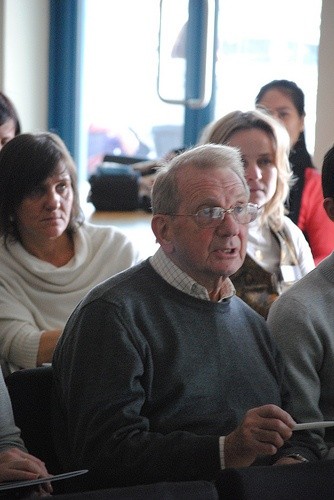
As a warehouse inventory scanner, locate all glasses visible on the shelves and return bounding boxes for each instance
[169,203,258,228]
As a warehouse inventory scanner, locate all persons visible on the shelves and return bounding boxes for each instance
[255,80,334,268]
[266,145,334,462]
[0,147,143,373]
[0,92,20,152]
[49,142,324,488]
[0,366,54,500]
[195,110,316,321]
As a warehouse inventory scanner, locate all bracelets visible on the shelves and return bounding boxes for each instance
[286,454,310,463]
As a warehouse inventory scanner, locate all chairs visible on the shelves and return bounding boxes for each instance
[5,369,334,500]
